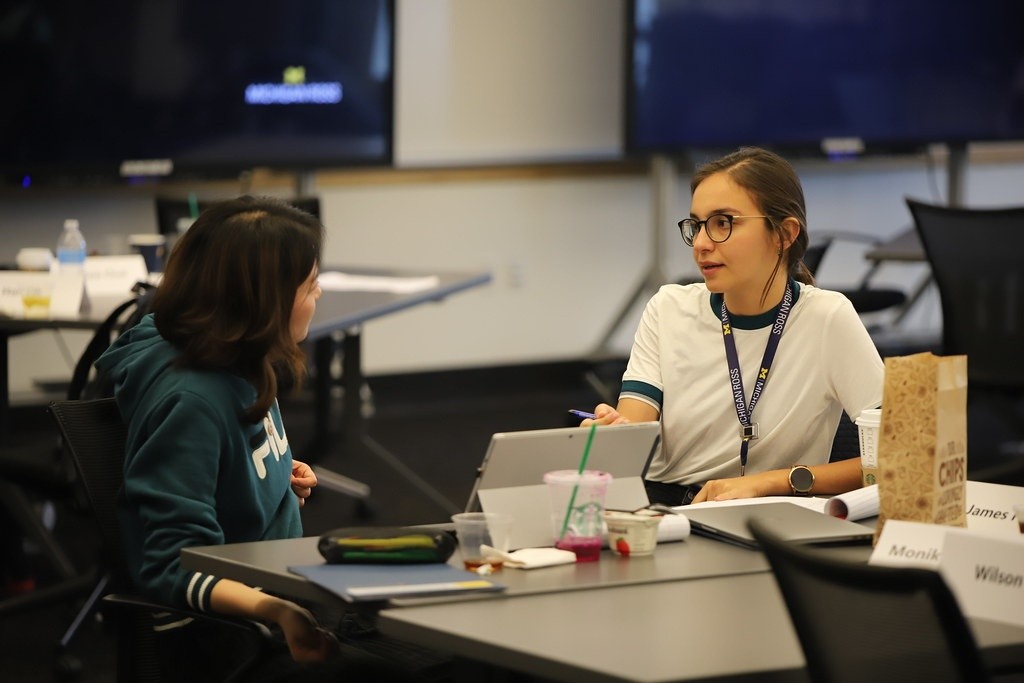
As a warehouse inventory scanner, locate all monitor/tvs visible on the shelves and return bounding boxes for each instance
[0,0,396,191]
[623,0,1024,171]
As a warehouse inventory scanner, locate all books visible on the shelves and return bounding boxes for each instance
[674,483,881,521]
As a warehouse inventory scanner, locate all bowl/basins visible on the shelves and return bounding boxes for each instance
[605,515,661,557]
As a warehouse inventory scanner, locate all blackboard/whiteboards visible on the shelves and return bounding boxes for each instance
[0,0,650,200]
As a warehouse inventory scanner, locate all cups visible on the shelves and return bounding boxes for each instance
[451,512,516,571]
[543,469,612,562]
[855,409,882,487]
[16,247,52,273]
[128,233,167,273]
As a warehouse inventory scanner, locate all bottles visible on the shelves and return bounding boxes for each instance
[57,218,85,274]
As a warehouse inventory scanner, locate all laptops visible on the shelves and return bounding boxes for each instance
[675,502,875,550]
[406,422,662,532]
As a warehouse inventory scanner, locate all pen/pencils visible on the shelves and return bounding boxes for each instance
[569,408,596,419]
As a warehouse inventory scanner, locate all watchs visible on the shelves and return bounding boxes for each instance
[789,464,815,497]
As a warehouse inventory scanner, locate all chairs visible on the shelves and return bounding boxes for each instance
[0,295,289,683]
[745,518,1024,683]
[575,230,910,405]
[902,194,1024,480]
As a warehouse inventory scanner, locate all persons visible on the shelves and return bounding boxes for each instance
[580,148,885,505]
[60,196,456,683]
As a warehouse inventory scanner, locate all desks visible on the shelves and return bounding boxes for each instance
[185,523,1023,683]
[862,232,941,334]
[1,249,491,472]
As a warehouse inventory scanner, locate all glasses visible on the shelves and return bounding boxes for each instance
[678,212,772,246]
